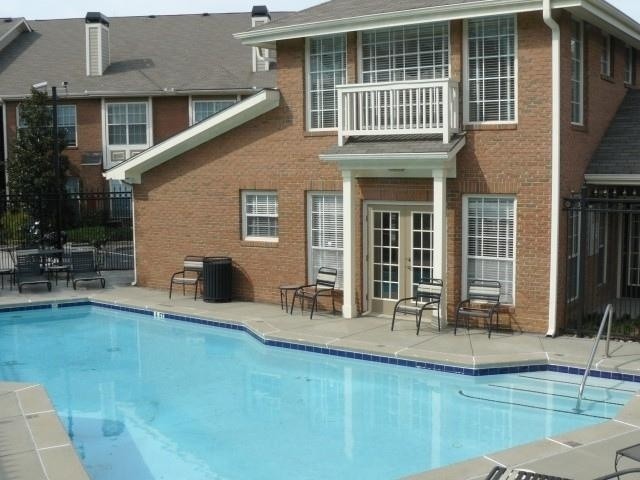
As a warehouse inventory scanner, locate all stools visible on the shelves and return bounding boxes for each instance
[278,284,307,312]
[610,441,640,480]
[483,463,570,479]
[47,263,71,289]
[0,268,14,291]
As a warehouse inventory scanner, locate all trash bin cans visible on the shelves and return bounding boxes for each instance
[203,257,232,303]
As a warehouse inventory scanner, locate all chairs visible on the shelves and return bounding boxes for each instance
[69,251,107,290]
[11,254,53,293]
[453,279,503,339]
[390,274,443,337]
[290,265,340,318]
[168,255,204,301]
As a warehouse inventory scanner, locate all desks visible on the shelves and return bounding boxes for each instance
[18,248,65,276]
